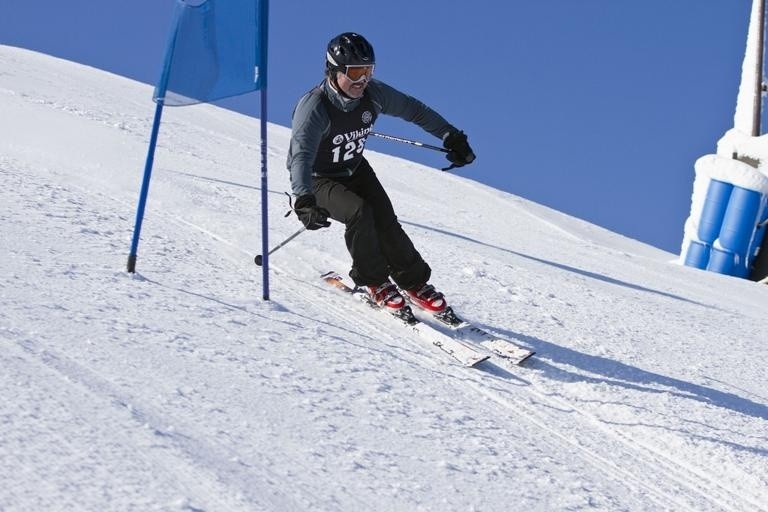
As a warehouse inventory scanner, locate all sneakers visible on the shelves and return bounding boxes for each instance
[367,282,406,310]
[402,282,447,311]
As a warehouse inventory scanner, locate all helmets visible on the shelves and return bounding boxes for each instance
[327,32,376,65]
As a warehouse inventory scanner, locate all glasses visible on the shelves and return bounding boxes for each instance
[345,65,374,83]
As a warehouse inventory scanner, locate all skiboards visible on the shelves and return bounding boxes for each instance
[314,258,536,368]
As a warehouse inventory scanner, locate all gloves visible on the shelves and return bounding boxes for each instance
[293,193,332,231]
[443,129,476,170]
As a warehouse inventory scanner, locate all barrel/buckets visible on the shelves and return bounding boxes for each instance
[706,245,754,278]
[683,239,712,269]
[698,176,735,241]
[718,185,768,257]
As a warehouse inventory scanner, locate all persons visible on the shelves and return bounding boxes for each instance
[286,32,475,314]
